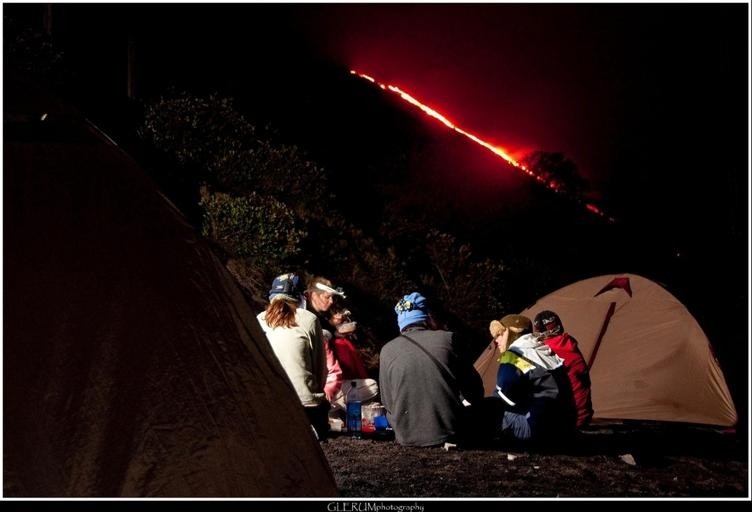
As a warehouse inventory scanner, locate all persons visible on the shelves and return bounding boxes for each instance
[378,291,485,450]
[465,316,575,454]
[256,274,332,440]
[531,311,595,429]
[301,275,355,332]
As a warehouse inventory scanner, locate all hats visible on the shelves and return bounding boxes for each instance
[534,309,564,336]
[393,290,432,330]
[488,314,533,350]
[266,271,304,306]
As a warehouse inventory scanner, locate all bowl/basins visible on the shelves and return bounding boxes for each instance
[337,322,357,333]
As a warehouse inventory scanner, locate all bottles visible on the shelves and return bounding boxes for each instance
[346,381,361,438]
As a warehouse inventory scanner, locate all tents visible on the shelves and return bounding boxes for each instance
[460,273,738,427]
